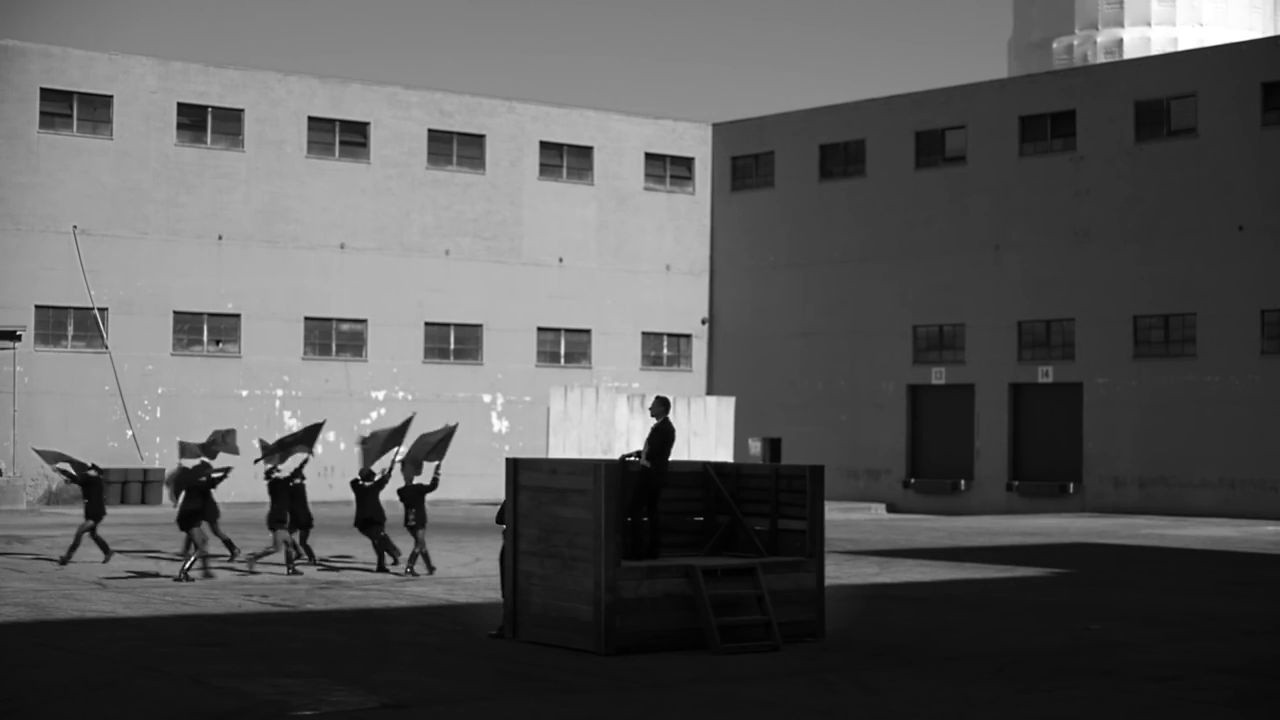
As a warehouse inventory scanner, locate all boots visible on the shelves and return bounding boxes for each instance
[178,555,197,582]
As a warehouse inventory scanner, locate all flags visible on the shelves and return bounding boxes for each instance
[165,466,229,504]
[253,422,323,464]
[180,428,240,459]
[359,416,414,468]
[398,425,457,476]
[35,449,88,474]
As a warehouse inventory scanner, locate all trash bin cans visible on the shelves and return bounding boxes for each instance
[101,468,126,505]
[143,468,165,505]
[123,468,144,505]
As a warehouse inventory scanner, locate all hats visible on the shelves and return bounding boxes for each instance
[358,467,375,482]
[264,466,279,475]
[88,462,104,474]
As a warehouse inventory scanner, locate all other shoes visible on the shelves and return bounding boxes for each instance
[101,550,114,563]
[376,567,390,573]
[287,567,304,576]
[428,566,436,576]
[228,548,240,561]
[392,561,399,566]
[405,567,419,577]
[202,570,213,578]
[246,554,255,571]
[60,555,67,564]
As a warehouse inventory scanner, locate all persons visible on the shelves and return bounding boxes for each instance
[350,468,401,572]
[488,500,506,638]
[620,396,674,560]
[245,457,316,575]
[59,463,113,565]
[176,460,241,582]
[396,462,440,576]
